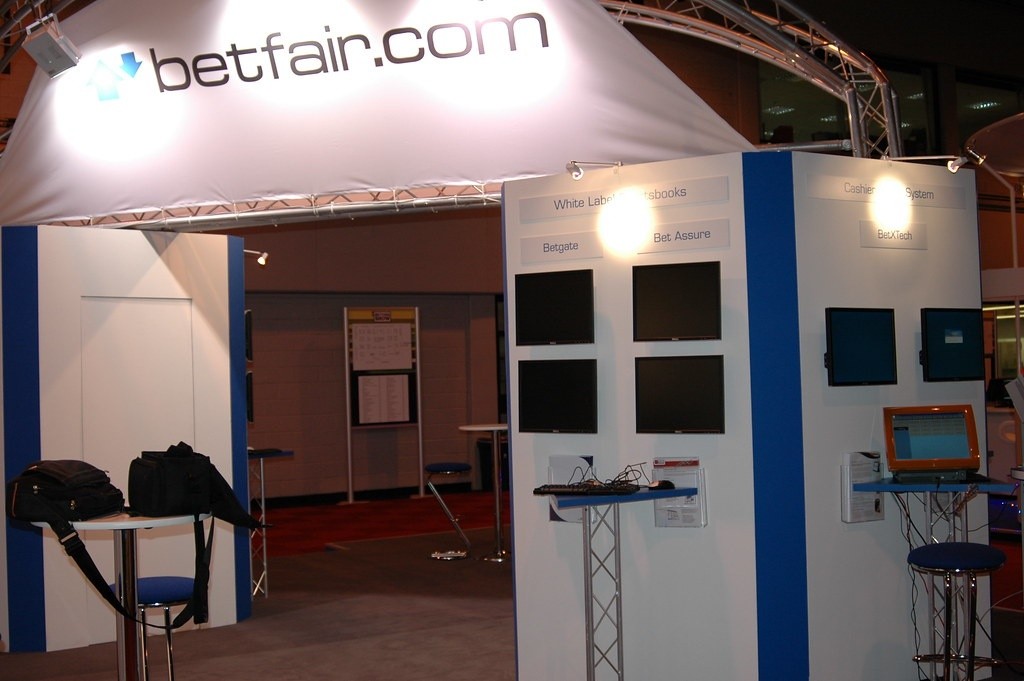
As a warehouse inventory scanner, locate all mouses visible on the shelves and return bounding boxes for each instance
[647,480,675,490]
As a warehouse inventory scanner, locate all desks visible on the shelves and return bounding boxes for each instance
[249,448,295,600]
[458,423,509,562]
[32,513,214,681]
[553,485,698,681]
[855,475,1016,681]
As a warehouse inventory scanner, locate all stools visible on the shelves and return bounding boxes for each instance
[422,462,472,559]
[907,541,1007,681]
[108,575,195,681]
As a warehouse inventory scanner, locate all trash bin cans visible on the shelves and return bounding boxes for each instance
[478,437,510,492]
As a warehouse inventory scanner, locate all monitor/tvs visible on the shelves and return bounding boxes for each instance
[635,355,725,435]
[632,261,722,341]
[515,269,595,346]
[986,378,1017,408]
[518,359,598,434]
[883,404,980,485]
[920,308,985,382]
[825,306,898,386]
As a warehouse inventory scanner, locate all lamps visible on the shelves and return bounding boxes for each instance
[565,161,623,180]
[23,13,83,79]
[884,148,968,173]
[243,250,269,266]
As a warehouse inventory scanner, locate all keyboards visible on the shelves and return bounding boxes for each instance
[533,484,641,495]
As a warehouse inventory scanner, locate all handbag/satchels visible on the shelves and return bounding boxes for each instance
[129,441,272,531]
[9,458,124,525]
